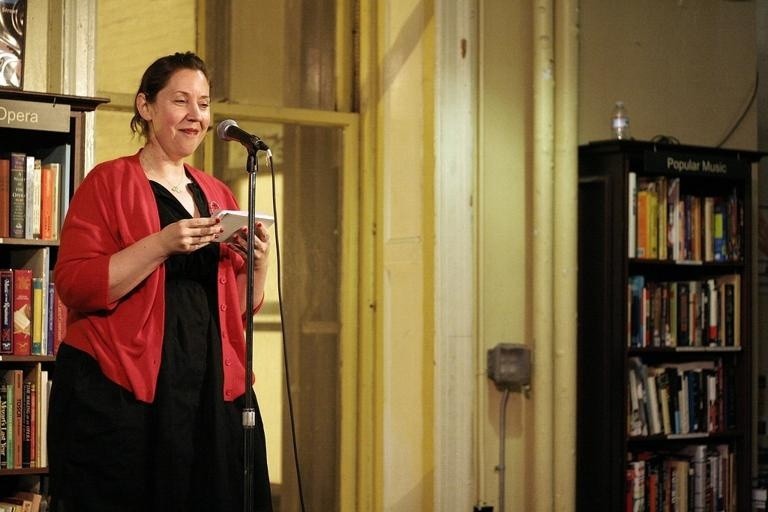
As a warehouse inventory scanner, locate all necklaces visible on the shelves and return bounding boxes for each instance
[141,150,185,193]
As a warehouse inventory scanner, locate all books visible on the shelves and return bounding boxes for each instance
[627,356,739,437]
[627,274,741,349]
[0,144,72,512]
[626,444,738,511]
[628,172,745,263]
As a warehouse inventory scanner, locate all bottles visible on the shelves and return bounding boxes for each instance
[610,101,630,139]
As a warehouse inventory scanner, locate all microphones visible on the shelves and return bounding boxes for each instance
[213,118,270,152]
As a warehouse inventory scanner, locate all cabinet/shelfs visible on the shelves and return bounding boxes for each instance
[578,139,764,512]
[0,88,109,511]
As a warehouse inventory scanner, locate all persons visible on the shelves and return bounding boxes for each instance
[46,51,271,512]
